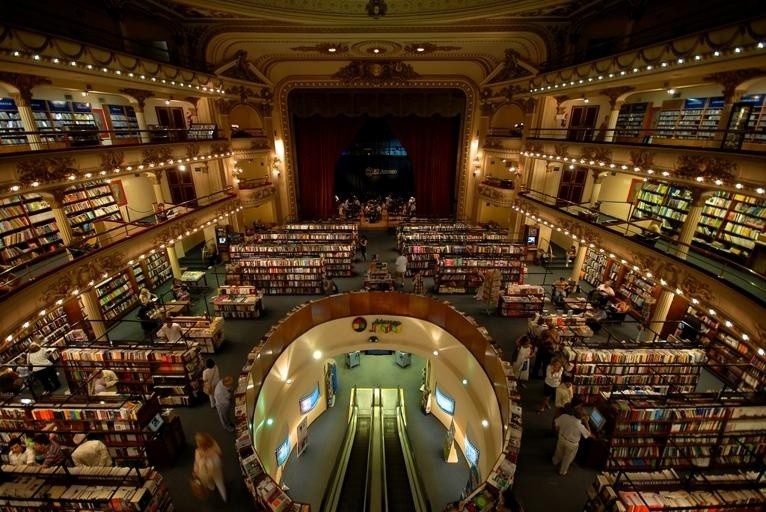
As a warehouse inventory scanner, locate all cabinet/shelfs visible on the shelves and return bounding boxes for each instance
[0,95,141,154]
[0,182,128,277]
[635,181,765,272]
[612,95,766,153]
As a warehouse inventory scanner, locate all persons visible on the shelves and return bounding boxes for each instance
[354,194,400,226]
[358,234,369,264]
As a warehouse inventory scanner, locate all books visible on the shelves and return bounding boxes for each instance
[1,102,357,511]
[396,93,765,512]
[361,252,394,293]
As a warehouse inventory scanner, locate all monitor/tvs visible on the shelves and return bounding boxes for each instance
[589,406,607,433]
